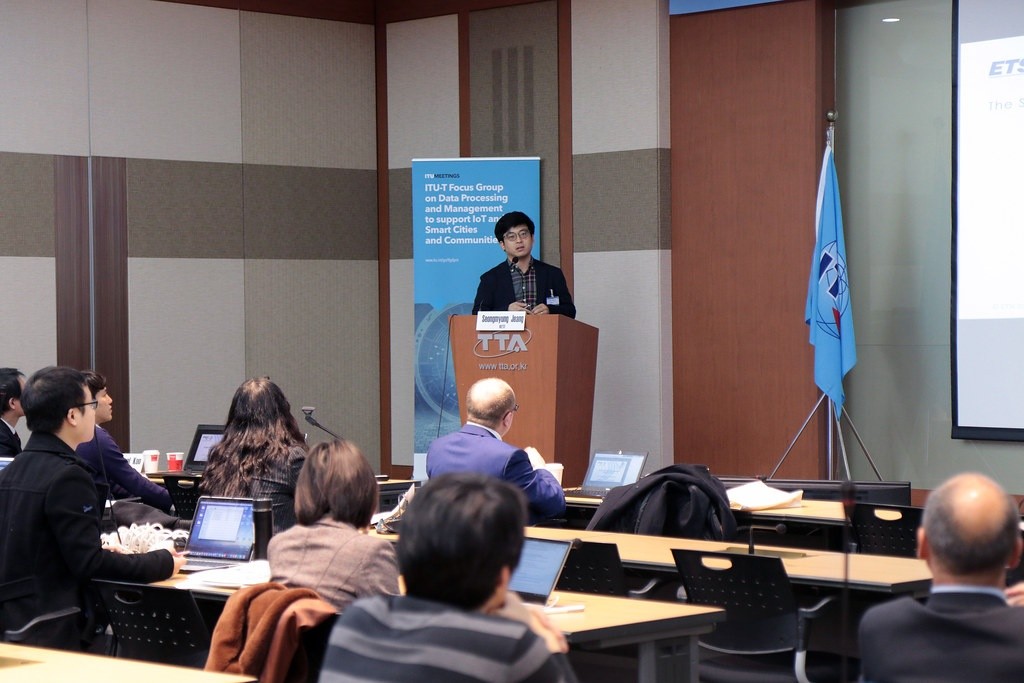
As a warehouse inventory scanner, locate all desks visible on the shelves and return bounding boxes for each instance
[105,538,724,683]
[378,525,933,683]
[0,642,259,683]
[147,471,421,510]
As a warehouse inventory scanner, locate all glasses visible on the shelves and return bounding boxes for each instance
[502,229,531,241]
[502,404,519,421]
[65,398,98,418]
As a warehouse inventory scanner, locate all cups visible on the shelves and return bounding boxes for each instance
[166,452,184,471]
[142,449,160,473]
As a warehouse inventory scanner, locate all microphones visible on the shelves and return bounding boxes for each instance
[479,257,519,311]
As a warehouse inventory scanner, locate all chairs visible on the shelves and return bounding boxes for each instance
[91,579,211,671]
[843,501,925,559]
[555,541,658,683]
[161,476,202,520]
[670,548,834,683]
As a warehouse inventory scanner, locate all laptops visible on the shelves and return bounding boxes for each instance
[174,496,257,572]
[563,451,650,499]
[506,537,573,606]
[183,424,229,477]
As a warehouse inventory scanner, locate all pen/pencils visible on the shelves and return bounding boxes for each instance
[177,551,190,556]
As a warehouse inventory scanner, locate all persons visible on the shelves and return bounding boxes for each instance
[472,211,577,319]
[317,473,577,683]
[0,366,187,651]
[199,377,309,538]
[857,472,1024,683]
[267,440,402,613]
[426,378,566,526]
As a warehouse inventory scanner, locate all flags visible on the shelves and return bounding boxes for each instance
[804,145,857,422]
